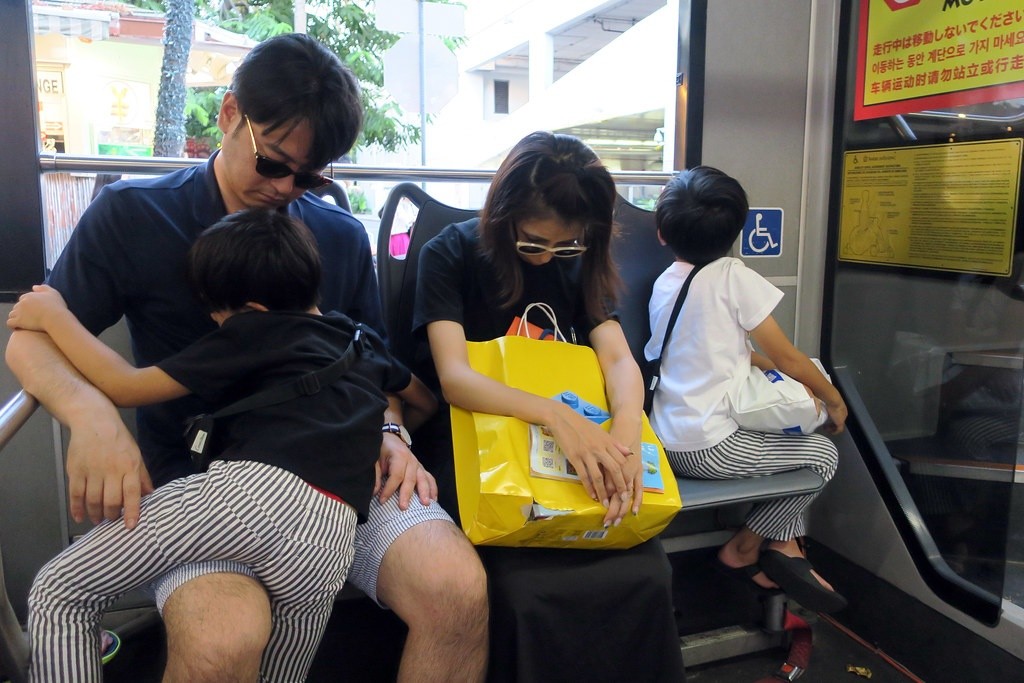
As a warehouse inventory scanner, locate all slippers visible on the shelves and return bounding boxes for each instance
[711,548,852,616]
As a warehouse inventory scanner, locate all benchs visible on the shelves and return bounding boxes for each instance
[376,183,825,667]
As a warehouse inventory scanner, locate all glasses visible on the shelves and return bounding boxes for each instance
[512,220,587,258]
[244,114,335,189]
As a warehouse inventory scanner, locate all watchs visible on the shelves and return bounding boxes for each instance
[382,422,411,451]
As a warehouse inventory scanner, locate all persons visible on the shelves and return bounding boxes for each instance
[4,208,435,682]
[412,130,684,683]
[645,166,848,616]
[3,29,492,682]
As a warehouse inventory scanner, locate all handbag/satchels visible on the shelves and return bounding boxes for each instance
[727,359,832,435]
[449,303,682,549]
